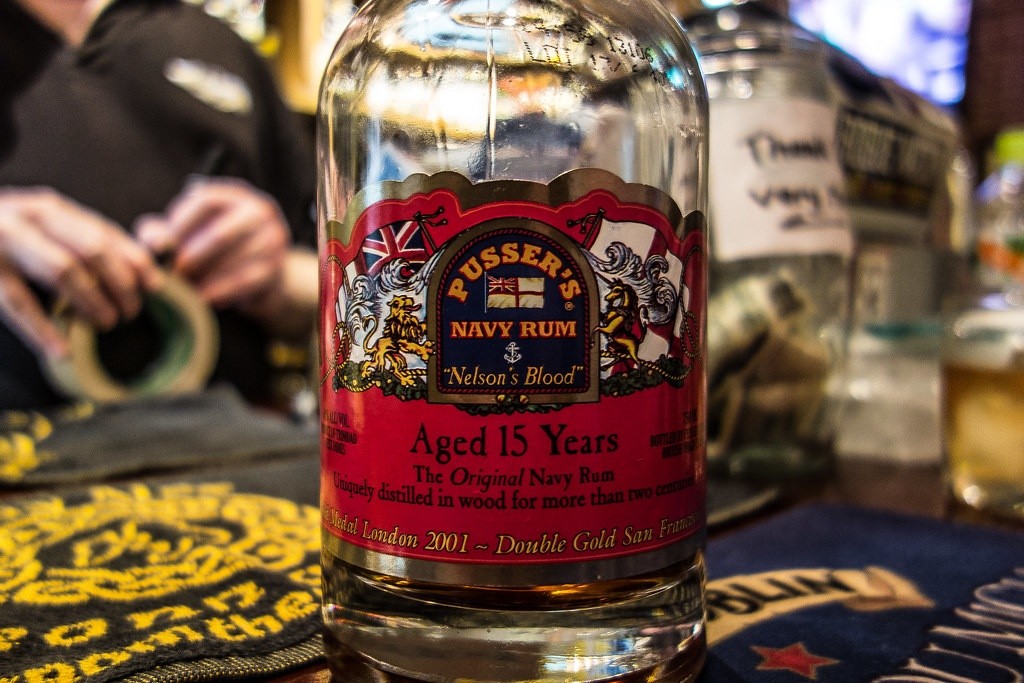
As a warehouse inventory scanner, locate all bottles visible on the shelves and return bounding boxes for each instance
[686,27,857,537]
[974,127,1024,308]
[315,0,720,683]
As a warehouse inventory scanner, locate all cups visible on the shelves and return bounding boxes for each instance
[940,303,1024,514]
[828,322,948,520]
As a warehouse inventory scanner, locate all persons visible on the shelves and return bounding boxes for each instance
[0,0,320,466]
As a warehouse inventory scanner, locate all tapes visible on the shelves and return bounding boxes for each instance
[44,270,222,410]
